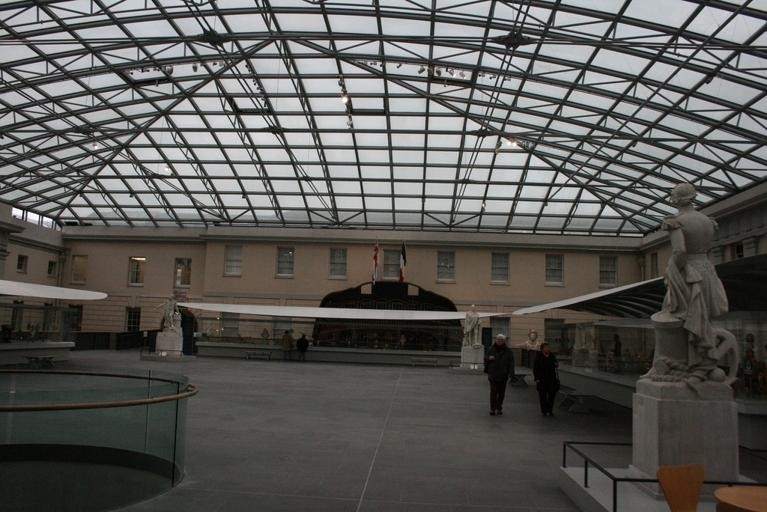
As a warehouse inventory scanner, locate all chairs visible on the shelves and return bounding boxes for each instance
[657,465,704,511]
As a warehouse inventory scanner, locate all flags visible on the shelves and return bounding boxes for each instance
[372,240,379,285]
[399,241,407,284]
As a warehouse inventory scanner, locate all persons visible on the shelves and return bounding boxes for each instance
[280,330,295,361]
[533,342,560,416]
[27,319,41,338]
[661,183,729,321]
[609,334,623,373]
[623,348,632,369]
[153,293,180,328]
[516,328,546,351]
[296,333,309,361]
[483,334,518,415]
[463,304,482,346]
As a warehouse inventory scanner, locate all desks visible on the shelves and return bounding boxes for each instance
[713,486,767,512]
[509,367,531,387]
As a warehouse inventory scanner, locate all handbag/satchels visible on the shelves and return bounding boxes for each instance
[556,362,560,391]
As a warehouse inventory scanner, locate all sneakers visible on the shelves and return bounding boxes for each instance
[543,412,554,417]
[489,410,503,416]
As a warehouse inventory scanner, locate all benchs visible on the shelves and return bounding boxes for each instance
[410,357,439,367]
[449,359,461,367]
[23,355,55,370]
[245,351,272,360]
